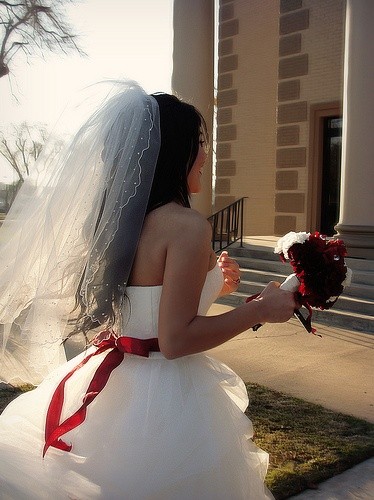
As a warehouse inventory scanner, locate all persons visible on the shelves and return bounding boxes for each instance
[1,93,301,500]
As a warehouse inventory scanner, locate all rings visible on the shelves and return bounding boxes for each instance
[232,277,241,283]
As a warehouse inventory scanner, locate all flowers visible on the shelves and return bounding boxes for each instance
[247,232,348,330]
